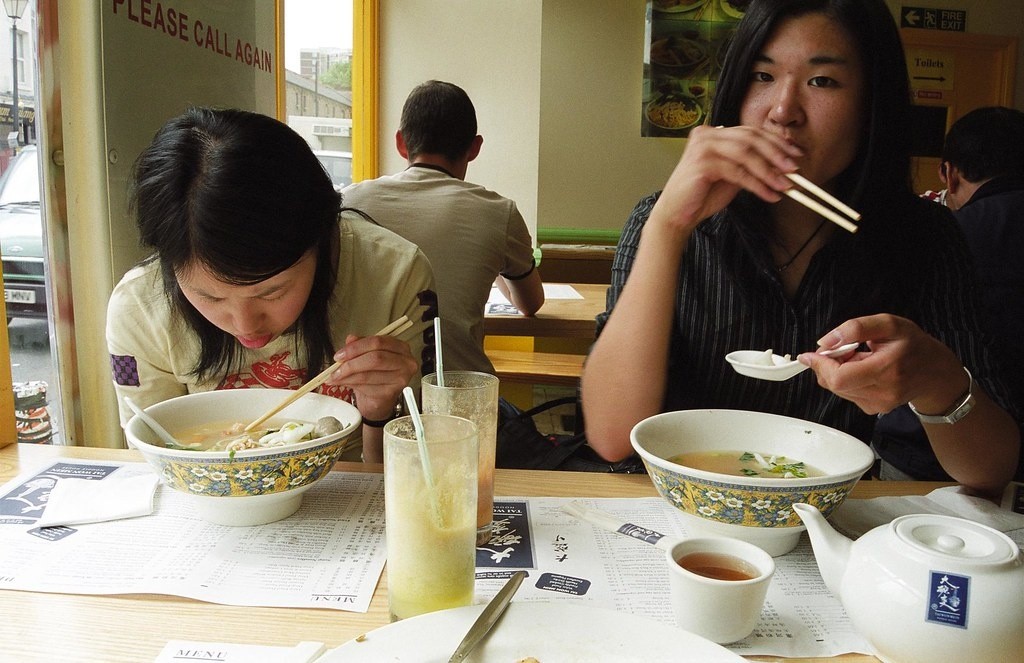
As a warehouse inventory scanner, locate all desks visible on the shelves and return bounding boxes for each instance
[483,282,612,340]
[540,242,618,285]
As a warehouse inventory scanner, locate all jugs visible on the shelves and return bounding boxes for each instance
[791,503,1024,663]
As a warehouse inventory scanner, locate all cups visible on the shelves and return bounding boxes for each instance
[421,371,500,547]
[665,535,775,645]
[383,414,479,623]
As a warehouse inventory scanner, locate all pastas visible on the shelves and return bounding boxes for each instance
[649,101,699,127]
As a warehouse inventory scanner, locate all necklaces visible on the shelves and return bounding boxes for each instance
[767,215,825,274]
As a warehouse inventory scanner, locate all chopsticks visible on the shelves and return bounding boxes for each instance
[684,57,710,80]
[694,0,710,21]
[717,125,861,234]
[245,316,413,432]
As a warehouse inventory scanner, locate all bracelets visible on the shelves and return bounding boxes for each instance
[362,401,404,427]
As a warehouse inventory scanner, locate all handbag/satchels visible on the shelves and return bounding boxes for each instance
[495,396,646,473]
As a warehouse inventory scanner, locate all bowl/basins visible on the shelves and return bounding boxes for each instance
[644,94,702,130]
[630,409,875,558]
[125,388,362,527]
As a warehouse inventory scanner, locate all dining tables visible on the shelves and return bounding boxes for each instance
[0,441,1024,663]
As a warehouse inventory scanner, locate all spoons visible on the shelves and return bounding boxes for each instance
[724,342,860,382]
[124,396,183,448]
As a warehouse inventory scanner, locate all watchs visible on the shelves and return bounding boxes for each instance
[906,364,977,424]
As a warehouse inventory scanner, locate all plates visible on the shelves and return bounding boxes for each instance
[720,0,746,19]
[652,0,706,13]
[689,84,707,97]
[312,601,751,663]
[650,38,706,67]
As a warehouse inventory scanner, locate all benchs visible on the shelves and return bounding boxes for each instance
[484,348,588,388]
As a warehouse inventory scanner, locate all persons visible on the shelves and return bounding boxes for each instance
[579,0,1024,495]
[339,81,545,453]
[106,108,439,463]
[937,106,1024,329]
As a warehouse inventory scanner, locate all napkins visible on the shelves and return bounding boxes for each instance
[830,485,1024,538]
[31,464,161,530]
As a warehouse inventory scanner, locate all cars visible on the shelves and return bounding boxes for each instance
[312,150,353,189]
[0,145,48,326]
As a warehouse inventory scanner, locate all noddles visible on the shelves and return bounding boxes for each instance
[159,420,323,454]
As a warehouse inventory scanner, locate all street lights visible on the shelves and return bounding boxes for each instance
[4,0,30,156]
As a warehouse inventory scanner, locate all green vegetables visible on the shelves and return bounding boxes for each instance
[736,451,808,480]
